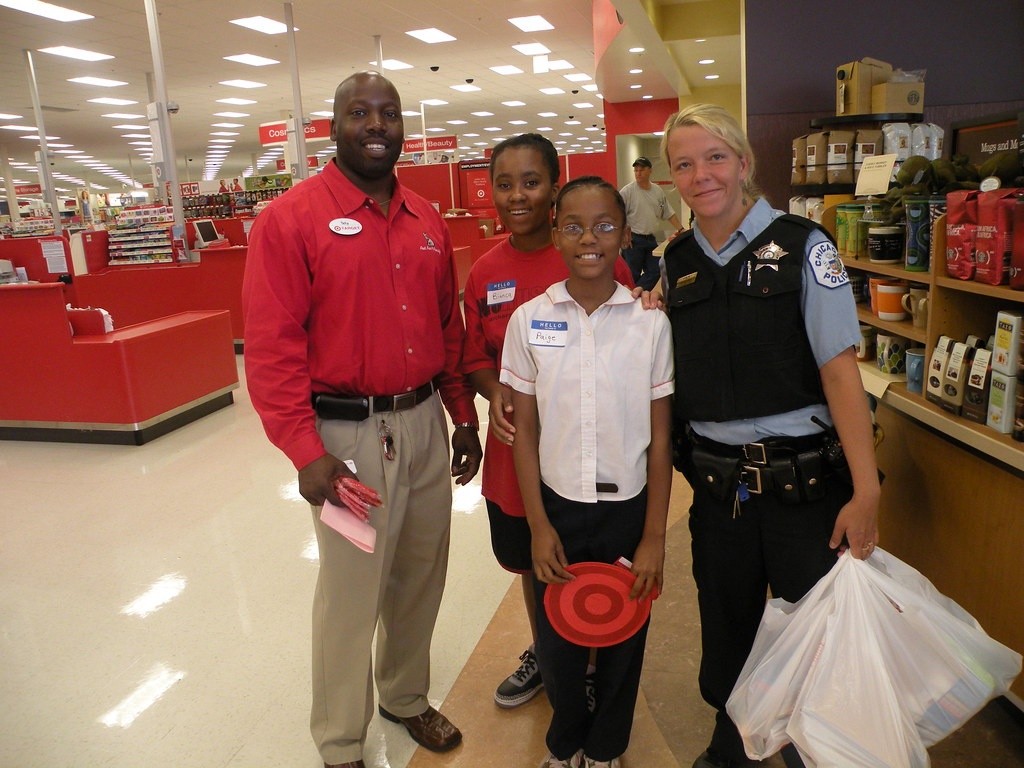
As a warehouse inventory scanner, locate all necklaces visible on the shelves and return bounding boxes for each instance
[378,199,391,205]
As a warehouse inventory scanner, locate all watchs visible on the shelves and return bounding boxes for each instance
[454,419,480,432]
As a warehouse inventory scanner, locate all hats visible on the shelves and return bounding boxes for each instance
[632,157,652,169]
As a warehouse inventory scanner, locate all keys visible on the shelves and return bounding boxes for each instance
[387,437,397,456]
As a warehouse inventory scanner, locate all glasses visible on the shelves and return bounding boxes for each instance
[557,222,621,241]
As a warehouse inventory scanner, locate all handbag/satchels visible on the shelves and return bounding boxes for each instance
[724,541,1022,768]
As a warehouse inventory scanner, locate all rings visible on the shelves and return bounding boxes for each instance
[862,540,874,550]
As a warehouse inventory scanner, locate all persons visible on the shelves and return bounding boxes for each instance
[463,131,632,708]
[240,69,483,768]
[496,177,676,768]
[619,157,687,295]
[80,191,93,225]
[232,178,243,191]
[630,102,881,768]
[258,176,290,188]
[218,180,230,192]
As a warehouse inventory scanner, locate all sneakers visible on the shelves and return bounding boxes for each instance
[540,745,620,768]
[493,642,543,707]
[583,672,598,714]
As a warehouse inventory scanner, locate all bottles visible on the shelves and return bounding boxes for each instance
[905,192,951,271]
[837,203,886,257]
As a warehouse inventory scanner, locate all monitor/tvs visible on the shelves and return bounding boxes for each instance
[193,219,220,246]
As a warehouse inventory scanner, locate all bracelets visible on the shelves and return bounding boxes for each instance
[678,226,684,232]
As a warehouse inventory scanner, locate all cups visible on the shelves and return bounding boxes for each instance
[869,226,903,262]
[844,273,930,392]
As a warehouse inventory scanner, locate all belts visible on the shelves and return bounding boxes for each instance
[315,377,440,412]
[695,440,795,494]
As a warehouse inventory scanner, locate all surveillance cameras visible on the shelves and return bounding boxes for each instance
[466,79,473,84]
[569,116,574,119]
[592,125,597,127]
[430,67,439,72]
[601,127,605,130]
[189,158,193,161]
[572,90,578,94]
[50,162,54,165]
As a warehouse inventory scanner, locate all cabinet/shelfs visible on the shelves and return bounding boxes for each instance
[108,223,173,266]
[822,188,1024,477]
[183,187,293,217]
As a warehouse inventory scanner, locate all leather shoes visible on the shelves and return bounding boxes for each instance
[691,744,734,768]
[379,702,462,751]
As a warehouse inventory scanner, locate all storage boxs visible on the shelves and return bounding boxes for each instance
[986,311,1022,434]
[835,57,924,113]
